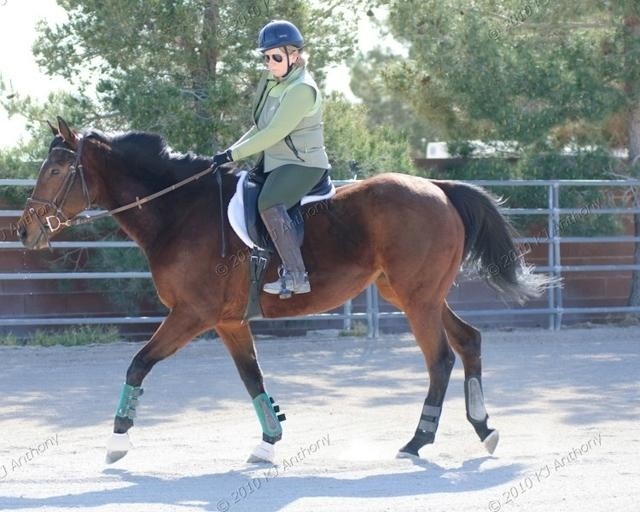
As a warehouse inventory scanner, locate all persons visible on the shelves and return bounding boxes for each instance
[211,20,331,294]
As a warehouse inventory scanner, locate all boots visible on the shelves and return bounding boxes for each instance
[260,203,312,294]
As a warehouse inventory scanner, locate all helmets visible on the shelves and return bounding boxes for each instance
[255,21,304,52]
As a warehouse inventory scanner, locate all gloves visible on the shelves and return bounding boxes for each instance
[211,150,233,174]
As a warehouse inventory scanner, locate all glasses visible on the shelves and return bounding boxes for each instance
[264,54,284,63]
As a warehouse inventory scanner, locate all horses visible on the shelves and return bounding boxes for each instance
[17,116,565,470]
